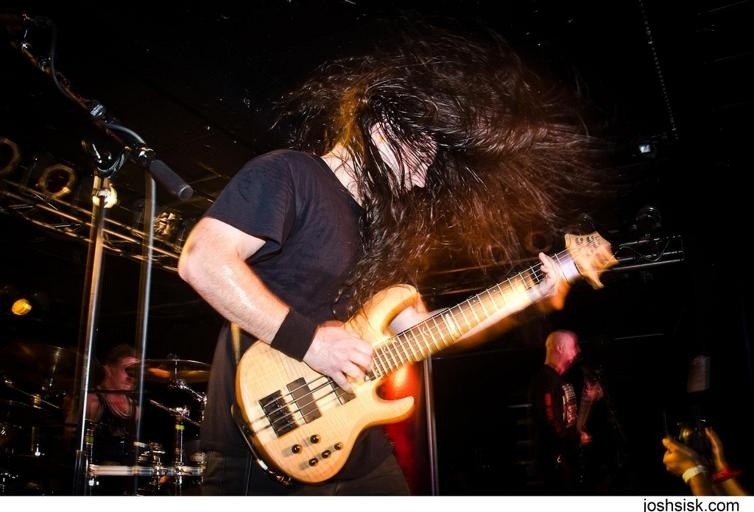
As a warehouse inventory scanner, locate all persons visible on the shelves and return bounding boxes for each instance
[174,36,603,493]
[525,329,603,496]
[661,422,748,495]
[83,344,160,496]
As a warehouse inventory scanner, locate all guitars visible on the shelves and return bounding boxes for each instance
[553,363,603,484]
[235,232,620,484]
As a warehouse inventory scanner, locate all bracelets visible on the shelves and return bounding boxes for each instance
[709,466,745,483]
[679,463,706,482]
[269,306,319,366]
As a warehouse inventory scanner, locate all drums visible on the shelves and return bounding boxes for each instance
[0,399,48,475]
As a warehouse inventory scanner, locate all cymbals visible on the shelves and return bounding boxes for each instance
[0,341,105,398]
[124,358,211,385]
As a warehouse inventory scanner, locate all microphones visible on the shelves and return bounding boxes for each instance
[148,160,194,202]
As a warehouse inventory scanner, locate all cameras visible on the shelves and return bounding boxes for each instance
[678,419,706,446]
